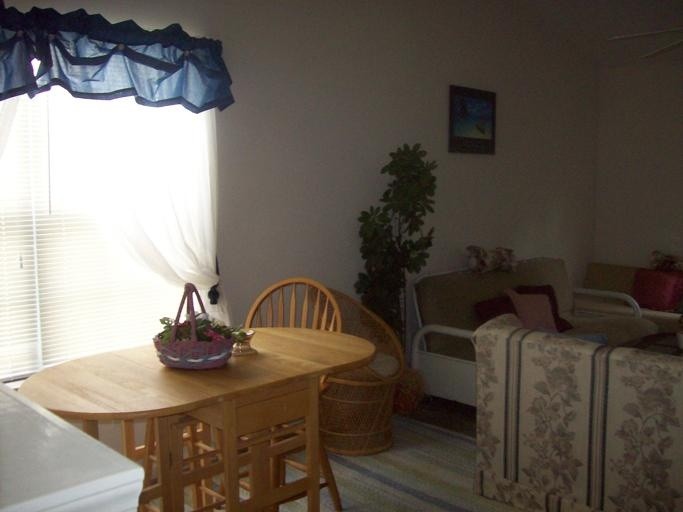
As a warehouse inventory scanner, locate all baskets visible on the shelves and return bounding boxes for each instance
[152,283,234,370]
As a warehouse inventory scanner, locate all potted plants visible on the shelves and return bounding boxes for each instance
[354,144,437,418]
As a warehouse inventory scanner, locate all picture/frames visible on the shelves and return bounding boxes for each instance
[450,83,498,154]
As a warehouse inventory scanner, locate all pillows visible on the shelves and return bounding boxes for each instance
[473,285,573,333]
[625,269,683,312]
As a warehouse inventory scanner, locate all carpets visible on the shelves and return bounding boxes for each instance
[146,420,524,512]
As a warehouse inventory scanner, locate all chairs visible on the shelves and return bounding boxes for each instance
[244,278,342,511]
[309,286,404,457]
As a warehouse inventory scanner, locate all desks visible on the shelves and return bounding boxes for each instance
[18,327,377,512]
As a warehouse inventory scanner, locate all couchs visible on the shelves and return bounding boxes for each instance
[470,314,683,512]
[575,263,683,355]
[411,257,659,409]
